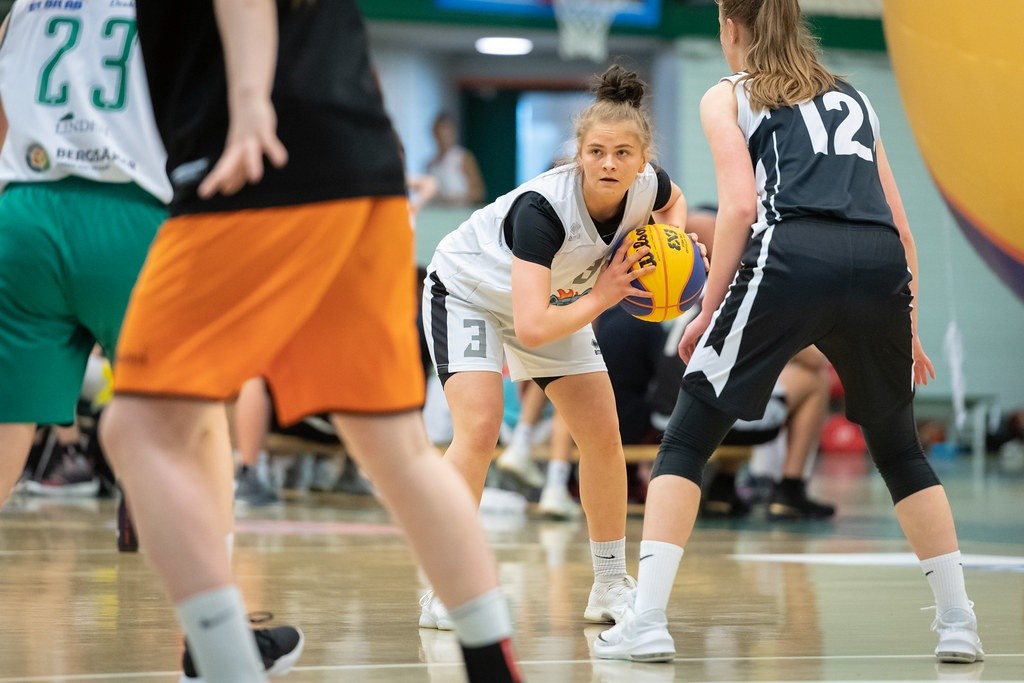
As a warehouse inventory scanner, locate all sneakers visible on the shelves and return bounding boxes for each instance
[418,588,456,630]
[592,589,676,663]
[584,575,637,624]
[920,600,986,662]
[181,625,304,682]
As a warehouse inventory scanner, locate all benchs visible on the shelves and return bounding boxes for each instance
[263,430,759,516]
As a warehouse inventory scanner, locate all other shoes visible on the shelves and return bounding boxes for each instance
[769,489,834,518]
[704,488,750,514]
[26,459,100,498]
[497,448,545,487]
[538,490,582,519]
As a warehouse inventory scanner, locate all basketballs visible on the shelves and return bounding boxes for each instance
[609,222,705,323]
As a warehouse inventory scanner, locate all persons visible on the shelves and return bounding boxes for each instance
[499,207,836,524]
[112,0,522,683]
[0,0,304,683]
[580,0,985,663]
[418,64,709,630]
[407,114,485,228]
